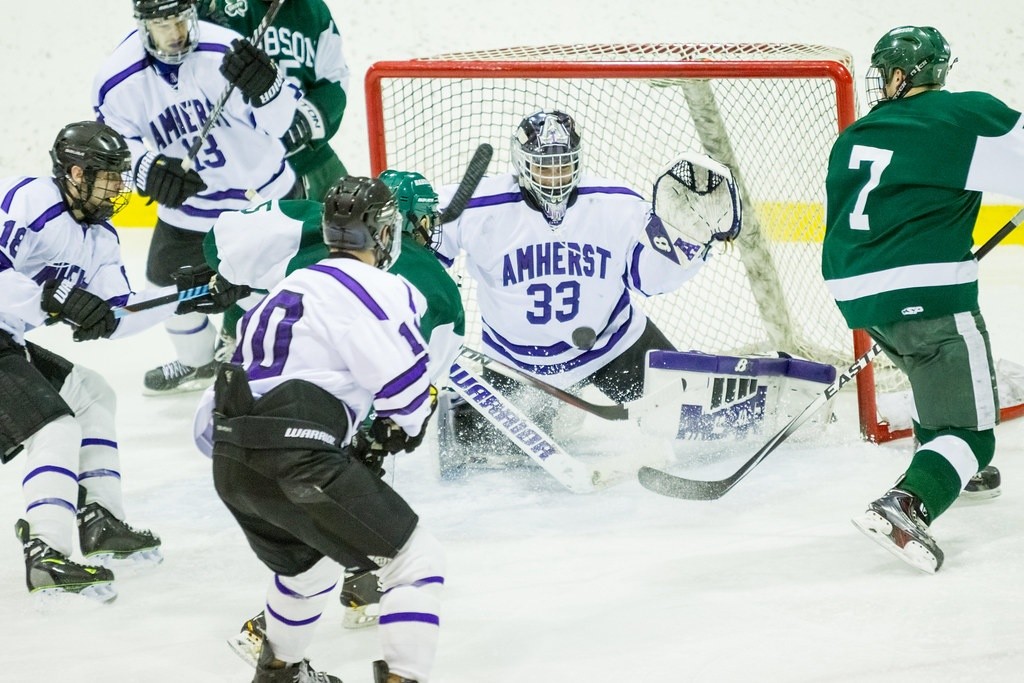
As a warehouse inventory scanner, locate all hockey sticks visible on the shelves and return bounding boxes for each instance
[634,215,1017,503]
[457,347,687,422]
[179,0,285,172]
[111,141,495,321]
[448,365,611,494]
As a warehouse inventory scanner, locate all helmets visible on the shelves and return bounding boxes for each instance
[510,111,583,225]
[133,0,200,65]
[379,170,444,253]
[322,175,404,271]
[866,25,950,109]
[51,120,134,224]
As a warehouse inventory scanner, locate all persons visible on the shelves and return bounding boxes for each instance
[211,174,456,683]
[429,111,742,478]
[821,26,1024,575]
[93,0,467,668]
[0,121,162,591]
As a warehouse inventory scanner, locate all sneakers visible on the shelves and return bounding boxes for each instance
[23,538,117,604]
[144,362,217,395]
[945,467,1001,511]
[852,489,945,572]
[82,501,162,566]
[240,611,343,683]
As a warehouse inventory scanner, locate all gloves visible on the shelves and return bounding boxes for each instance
[341,436,383,477]
[219,38,284,109]
[39,278,121,343]
[280,110,312,153]
[170,263,250,316]
[368,384,440,455]
[132,151,207,210]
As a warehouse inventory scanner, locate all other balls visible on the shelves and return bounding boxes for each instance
[573,327,598,351]
